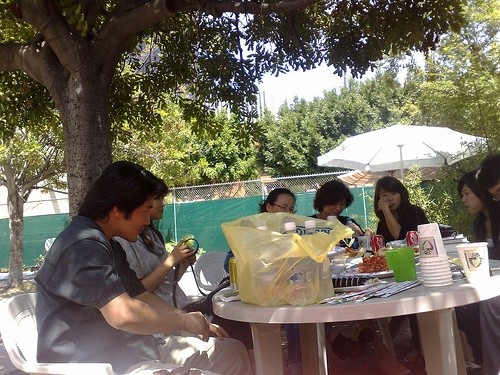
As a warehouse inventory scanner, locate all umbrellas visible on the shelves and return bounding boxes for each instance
[317,124,494,185]
[337,167,444,186]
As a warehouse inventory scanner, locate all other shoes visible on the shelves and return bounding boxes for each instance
[462,359,482,374]
[373,353,409,375]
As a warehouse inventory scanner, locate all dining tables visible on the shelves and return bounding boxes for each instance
[212,259,500,375]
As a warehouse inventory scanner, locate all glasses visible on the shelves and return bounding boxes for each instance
[490,194,500,204]
[271,202,300,214]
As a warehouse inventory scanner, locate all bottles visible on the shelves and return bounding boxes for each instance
[326,216,342,250]
[303,220,335,304]
[346,217,358,249]
[282,222,310,306]
[250,225,281,307]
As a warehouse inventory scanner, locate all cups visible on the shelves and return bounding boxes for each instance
[417,223,454,287]
[385,246,417,282]
[358,235,371,249]
[455,242,490,283]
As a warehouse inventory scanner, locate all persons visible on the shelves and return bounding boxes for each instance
[474,150,500,375]
[35,160,255,375]
[223,188,367,375]
[114,177,290,375]
[368,176,429,364]
[457,170,500,369]
[306,180,412,375]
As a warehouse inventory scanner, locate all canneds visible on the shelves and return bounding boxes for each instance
[406,231,419,246]
[228,256,238,290]
[371,234,384,253]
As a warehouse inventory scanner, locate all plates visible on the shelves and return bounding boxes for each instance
[330,269,394,279]
[332,282,382,293]
[351,256,365,265]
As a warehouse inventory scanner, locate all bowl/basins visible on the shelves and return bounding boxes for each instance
[329,257,346,274]
[386,239,406,248]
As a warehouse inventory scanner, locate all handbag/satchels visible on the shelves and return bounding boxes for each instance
[222,211,354,305]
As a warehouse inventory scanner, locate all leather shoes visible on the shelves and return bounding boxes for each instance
[329,335,365,361]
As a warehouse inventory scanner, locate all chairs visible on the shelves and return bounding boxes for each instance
[1,293,114,375]
[193,250,233,297]
